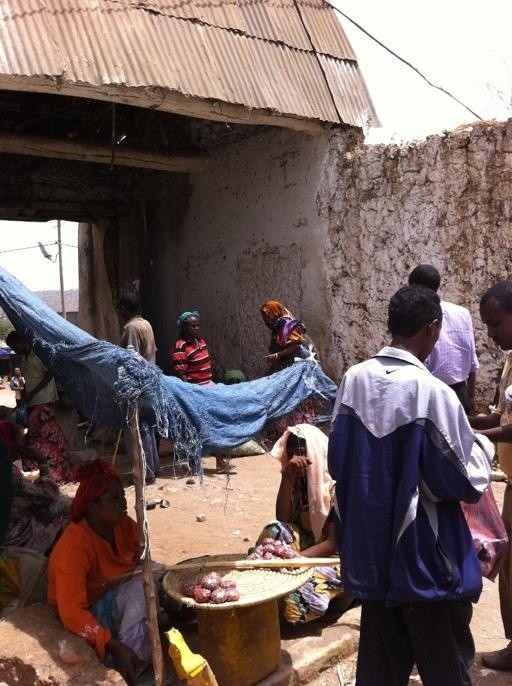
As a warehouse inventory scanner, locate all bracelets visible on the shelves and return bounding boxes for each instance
[275,353,278,359]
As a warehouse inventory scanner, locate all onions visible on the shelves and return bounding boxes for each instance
[183,571,240,603]
[240,537,299,570]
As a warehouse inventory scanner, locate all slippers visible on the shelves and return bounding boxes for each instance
[215,463,234,474]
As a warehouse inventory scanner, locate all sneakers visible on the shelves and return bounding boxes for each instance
[482,645,512,671]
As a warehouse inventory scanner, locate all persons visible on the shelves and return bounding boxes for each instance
[409,265,479,416]
[46,461,173,686]
[259,300,315,452]
[117,294,159,485]
[11,363,28,406]
[465,282,512,673]
[258,424,343,626]
[173,311,237,476]
[6,330,59,415]
[327,281,498,685]
